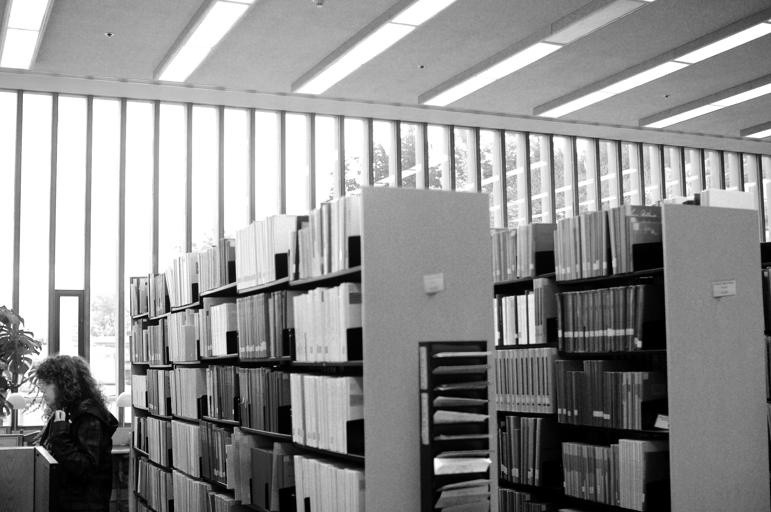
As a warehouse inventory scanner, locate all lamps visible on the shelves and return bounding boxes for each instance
[118,393,131,429]
[6,394,26,430]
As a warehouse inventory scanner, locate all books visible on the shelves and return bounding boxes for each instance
[490,223,557,512]
[761,266,771,338]
[131,366,235,511]
[288,193,365,512]
[231,214,304,512]
[553,205,672,512]
[131,237,235,319]
[132,303,237,365]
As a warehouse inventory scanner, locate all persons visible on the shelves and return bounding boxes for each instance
[37,353,116,510]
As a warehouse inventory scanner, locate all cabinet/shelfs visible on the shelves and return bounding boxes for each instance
[131,190,492,512]
[492,206,770,512]
